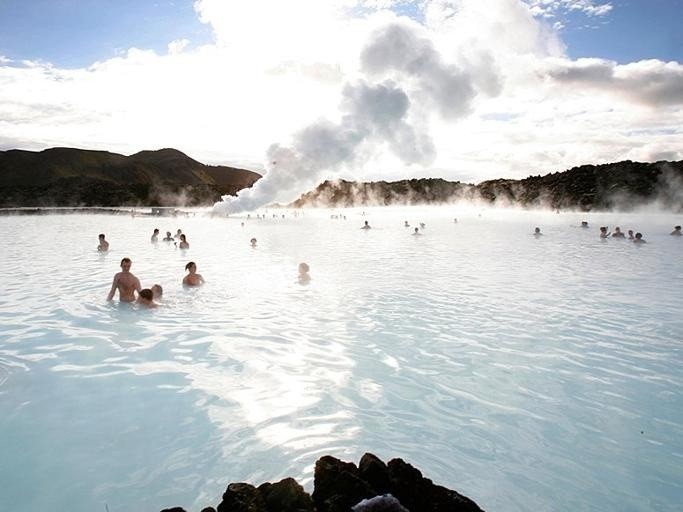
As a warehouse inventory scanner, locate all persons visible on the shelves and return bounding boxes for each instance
[170,207,196,220]
[151,228,189,250]
[297,262,311,286]
[182,262,205,288]
[669,226,683,237]
[97,234,109,252]
[138,289,159,309]
[532,227,543,236]
[240,209,427,236]
[569,203,648,243]
[250,239,258,246]
[152,285,163,302]
[106,258,142,302]
